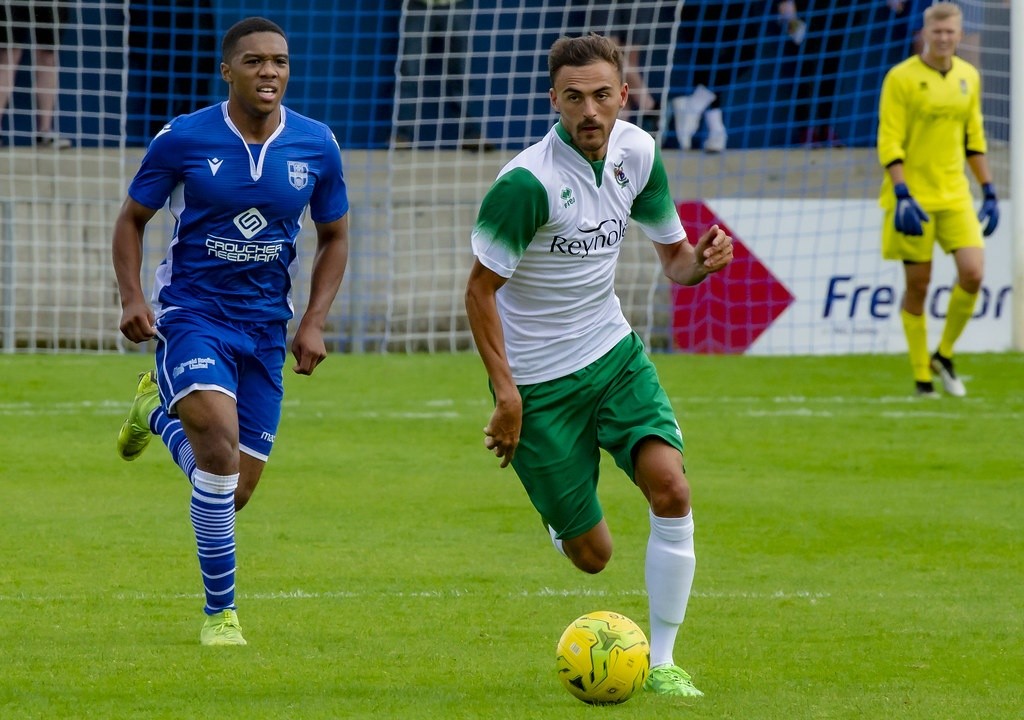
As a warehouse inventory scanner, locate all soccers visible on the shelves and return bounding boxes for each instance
[555,609,650,704]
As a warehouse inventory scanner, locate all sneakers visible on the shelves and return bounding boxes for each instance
[115,369,163,461]
[642,662,704,697]
[199,608,248,647]
[914,352,967,400]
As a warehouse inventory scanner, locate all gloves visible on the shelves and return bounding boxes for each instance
[893,183,929,236]
[977,182,1000,236]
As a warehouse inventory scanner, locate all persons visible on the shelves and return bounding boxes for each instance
[587,0,661,123]
[110,18,349,646]
[672,1,765,154]
[465,35,733,697]
[876,2,1001,401]
[912,0,987,74]
[388,0,494,151]
[0,0,71,150]
[776,0,903,149]
[130,0,216,115]
